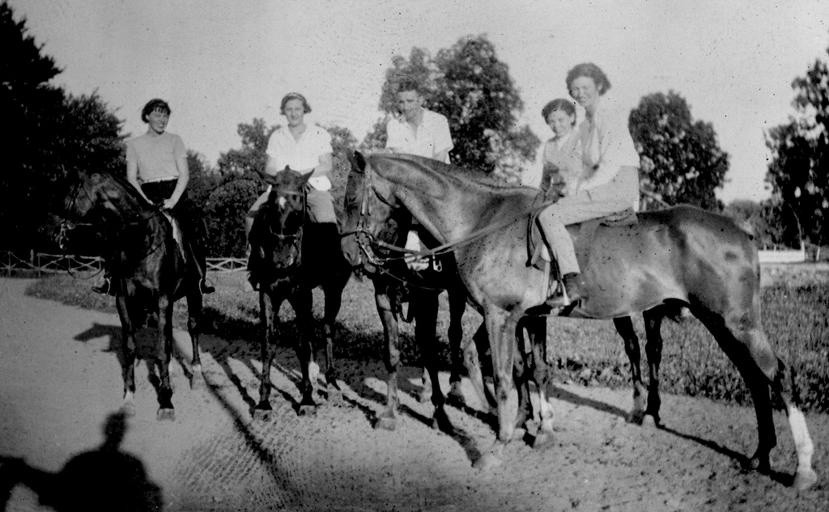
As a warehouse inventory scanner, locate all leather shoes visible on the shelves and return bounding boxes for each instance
[91,278,116,297]
[546,272,590,308]
[190,277,215,295]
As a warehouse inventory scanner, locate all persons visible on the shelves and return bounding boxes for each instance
[244,90,338,242]
[91,98,215,294]
[538,98,577,191]
[534,61,640,306]
[384,80,454,165]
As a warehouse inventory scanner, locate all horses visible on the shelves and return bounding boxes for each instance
[340,147,819,494]
[247,164,363,422]
[48,161,204,416]
